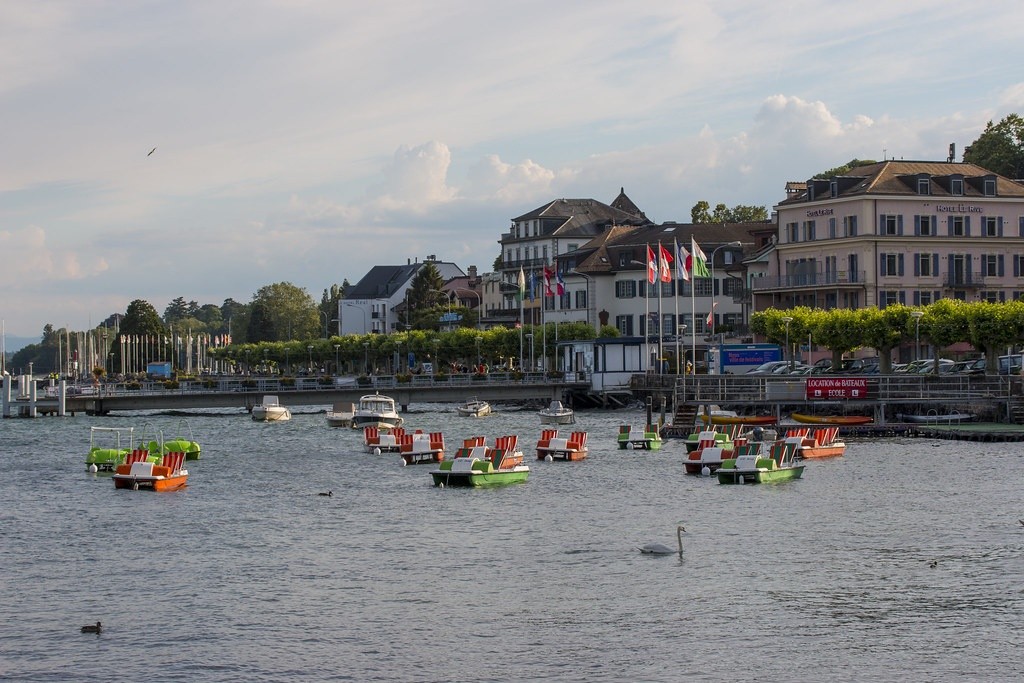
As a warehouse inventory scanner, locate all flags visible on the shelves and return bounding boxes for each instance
[527,263,539,304]
[544,268,554,298]
[659,242,673,283]
[647,244,658,284]
[517,266,525,302]
[555,259,566,296]
[675,238,692,282]
[692,236,711,278]
[120,335,232,344]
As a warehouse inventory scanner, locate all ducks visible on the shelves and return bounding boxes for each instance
[81,622,103,633]
[319,491,332,496]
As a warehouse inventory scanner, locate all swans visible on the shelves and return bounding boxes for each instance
[635,525,687,553]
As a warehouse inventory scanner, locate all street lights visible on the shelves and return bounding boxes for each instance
[780,317,794,375]
[711,240,741,349]
[629,259,662,337]
[428,288,451,333]
[474,336,484,373]
[456,287,482,332]
[678,323,687,373]
[910,312,923,375]
[228,261,404,391]
[499,281,524,326]
[525,334,534,372]
[432,339,441,373]
[566,271,596,339]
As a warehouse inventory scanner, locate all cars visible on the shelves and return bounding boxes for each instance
[743,354,1024,374]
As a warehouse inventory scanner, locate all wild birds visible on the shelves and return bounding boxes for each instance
[147,147,156,156]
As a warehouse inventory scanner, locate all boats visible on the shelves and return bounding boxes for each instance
[85,420,201,490]
[325,411,353,428]
[429,435,530,488]
[536,400,574,425]
[617,424,663,449]
[791,413,874,425]
[352,390,404,430]
[894,412,978,423]
[252,404,292,423]
[535,429,588,460]
[365,427,406,453]
[699,414,778,425]
[457,395,489,417]
[400,429,445,466]
[685,424,846,484]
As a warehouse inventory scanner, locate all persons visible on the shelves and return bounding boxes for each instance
[289,360,327,373]
[450,361,489,374]
